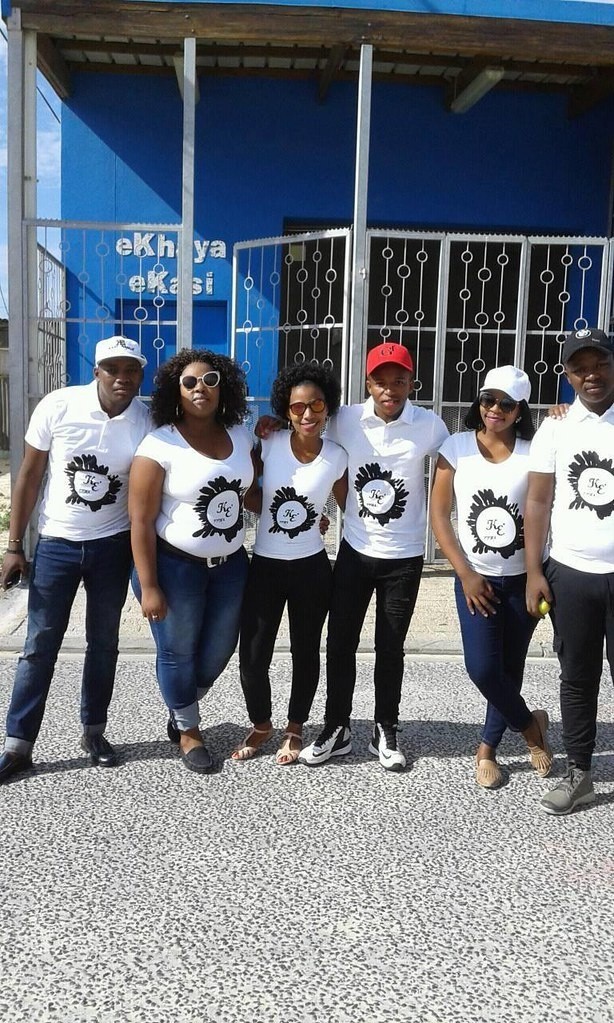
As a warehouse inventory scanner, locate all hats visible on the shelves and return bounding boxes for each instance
[480,365,532,403]
[366,342,414,378]
[95,335,147,369]
[561,328,613,363]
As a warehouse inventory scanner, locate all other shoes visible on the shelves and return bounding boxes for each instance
[525,709,553,778]
[167,716,181,745]
[178,729,215,774]
[476,752,504,788]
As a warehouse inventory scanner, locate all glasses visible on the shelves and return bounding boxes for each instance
[177,371,221,392]
[287,398,330,417]
[479,393,520,414]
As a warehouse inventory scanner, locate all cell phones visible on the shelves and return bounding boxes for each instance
[6,569,22,591]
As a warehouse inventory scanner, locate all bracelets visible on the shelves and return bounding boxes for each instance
[7,548,24,553]
[10,540,23,543]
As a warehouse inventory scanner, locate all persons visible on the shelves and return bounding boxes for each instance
[430,366,570,787]
[232,362,349,763]
[520,328,614,815]
[129,350,330,773]
[254,342,450,770]
[0,337,157,782]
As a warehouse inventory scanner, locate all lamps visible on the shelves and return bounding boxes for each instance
[450,54,505,114]
[289,242,307,261]
[171,51,201,105]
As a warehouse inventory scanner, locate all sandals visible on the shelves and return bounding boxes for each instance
[275,732,303,765]
[230,725,276,759]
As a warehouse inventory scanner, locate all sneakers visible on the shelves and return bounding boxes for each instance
[368,722,409,770]
[297,723,354,766]
[539,759,595,815]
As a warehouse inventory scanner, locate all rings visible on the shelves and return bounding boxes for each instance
[152,615,158,619]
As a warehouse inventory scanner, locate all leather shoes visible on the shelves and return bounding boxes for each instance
[0,750,32,783]
[80,733,116,767]
